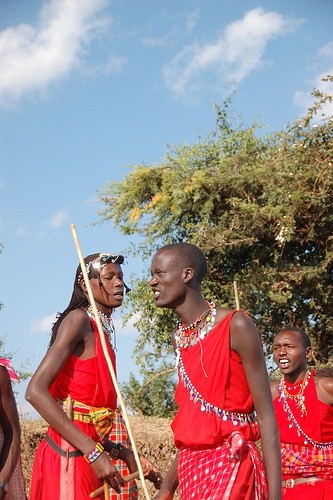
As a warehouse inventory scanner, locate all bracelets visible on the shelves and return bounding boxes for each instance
[84,443,105,465]
[110,442,124,462]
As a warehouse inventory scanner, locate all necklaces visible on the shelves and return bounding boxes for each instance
[86,304,118,338]
[175,299,217,345]
[277,371,311,419]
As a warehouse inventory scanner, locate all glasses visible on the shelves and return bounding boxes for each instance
[99,255,124,265]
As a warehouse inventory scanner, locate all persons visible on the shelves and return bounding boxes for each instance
[24,252,163,500]
[268,329,333,500]
[0,357,28,500]
[150,242,282,500]
